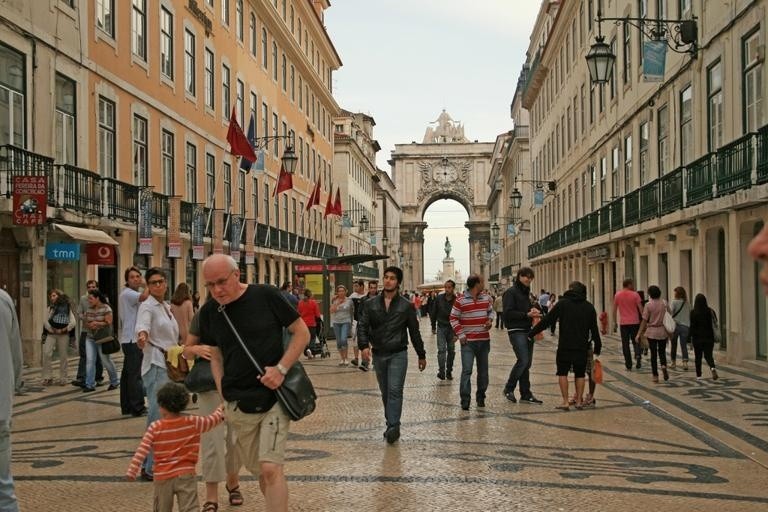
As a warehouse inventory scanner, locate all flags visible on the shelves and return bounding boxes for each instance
[238,112,256,175]
[334,187,343,217]
[324,186,336,220]
[305,176,321,211]
[225,106,257,163]
[272,164,292,198]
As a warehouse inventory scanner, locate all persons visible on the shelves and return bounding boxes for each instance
[747,221,768,299]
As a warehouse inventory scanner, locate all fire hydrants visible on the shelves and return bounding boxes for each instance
[599,311,608,334]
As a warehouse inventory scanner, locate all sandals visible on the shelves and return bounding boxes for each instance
[554,398,596,411]
[203,500,218,512]
[226,484,244,505]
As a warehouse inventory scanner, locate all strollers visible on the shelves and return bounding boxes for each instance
[306,317,332,359]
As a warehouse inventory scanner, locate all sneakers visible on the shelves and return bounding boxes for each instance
[476,399,485,406]
[437,373,445,380]
[519,397,544,405]
[339,359,369,371]
[137,408,149,417]
[446,371,452,380]
[504,389,518,403]
[384,425,400,443]
[42,378,118,393]
[626,349,704,382]
[462,401,469,409]
[307,349,314,359]
[711,367,718,380]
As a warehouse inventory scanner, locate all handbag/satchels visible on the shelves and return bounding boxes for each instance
[591,359,602,384]
[351,320,357,339]
[273,361,317,422]
[186,354,217,395]
[640,327,649,348]
[93,324,115,345]
[662,311,677,334]
[163,350,189,383]
[533,316,544,342]
[712,318,722,343]
[102,336,120,354]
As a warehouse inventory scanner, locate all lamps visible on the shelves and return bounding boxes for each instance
[583,14,700,84]
[507,174,556,207]
[337,206,368,232]
[489,212,521,236]
[243,133,295,177]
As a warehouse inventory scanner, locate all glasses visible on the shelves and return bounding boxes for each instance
[204,270,236,289]
